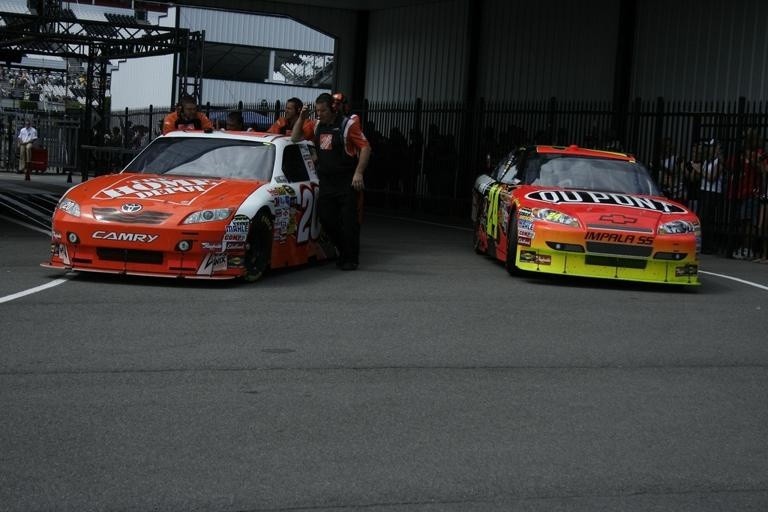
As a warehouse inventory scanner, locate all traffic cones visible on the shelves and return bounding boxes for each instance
[24,169,30,185]
[66,174,73,188]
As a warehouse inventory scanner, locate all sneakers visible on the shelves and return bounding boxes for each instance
[336,257,343,265]
[342,259,357,270]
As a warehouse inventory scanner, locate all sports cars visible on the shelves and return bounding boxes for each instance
[469,145,704,294]
[40,129,364,285]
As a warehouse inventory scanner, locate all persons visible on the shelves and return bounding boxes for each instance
[329,90,366,227]
[218,111,255,133]
[647,127,767,268]
[162,94,215,133]
[288,92,373,271]
[98,114,149,176]
[174,102,182,114]
[17,120,38,174]
[374,122,566,202]
[266,97,317,142]
